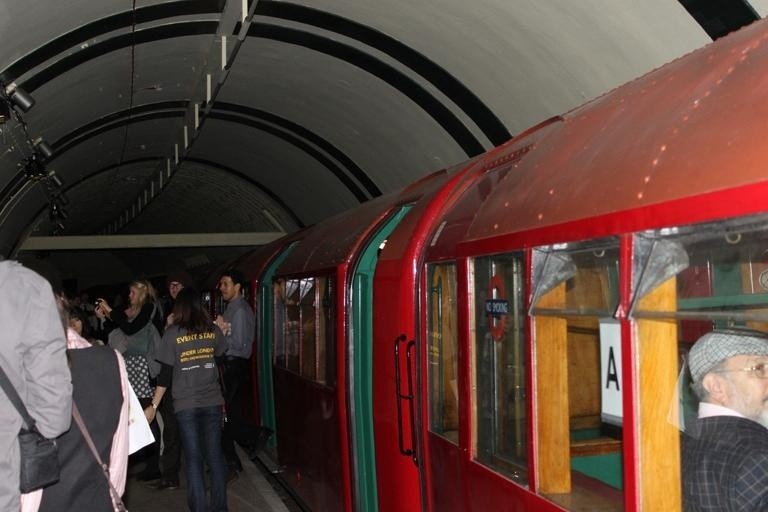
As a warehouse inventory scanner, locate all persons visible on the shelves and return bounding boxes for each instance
[680,327,767,510]
[66,271,255,512]
[0,260,73,512]
[17,288,129,512]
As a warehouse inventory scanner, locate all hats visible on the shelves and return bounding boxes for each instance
[688,325,768,381]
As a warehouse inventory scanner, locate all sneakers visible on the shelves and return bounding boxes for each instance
[144,471,179,490]
[227,466,244,484]
[130,472,161,482]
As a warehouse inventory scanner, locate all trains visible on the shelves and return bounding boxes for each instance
[75,15,768,512]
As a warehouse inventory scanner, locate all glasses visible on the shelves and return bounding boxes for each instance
[712,362,768,379]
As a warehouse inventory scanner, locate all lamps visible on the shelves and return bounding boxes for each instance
[0,79,70,235]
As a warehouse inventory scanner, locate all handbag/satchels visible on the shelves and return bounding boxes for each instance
[19,429,61,494]
